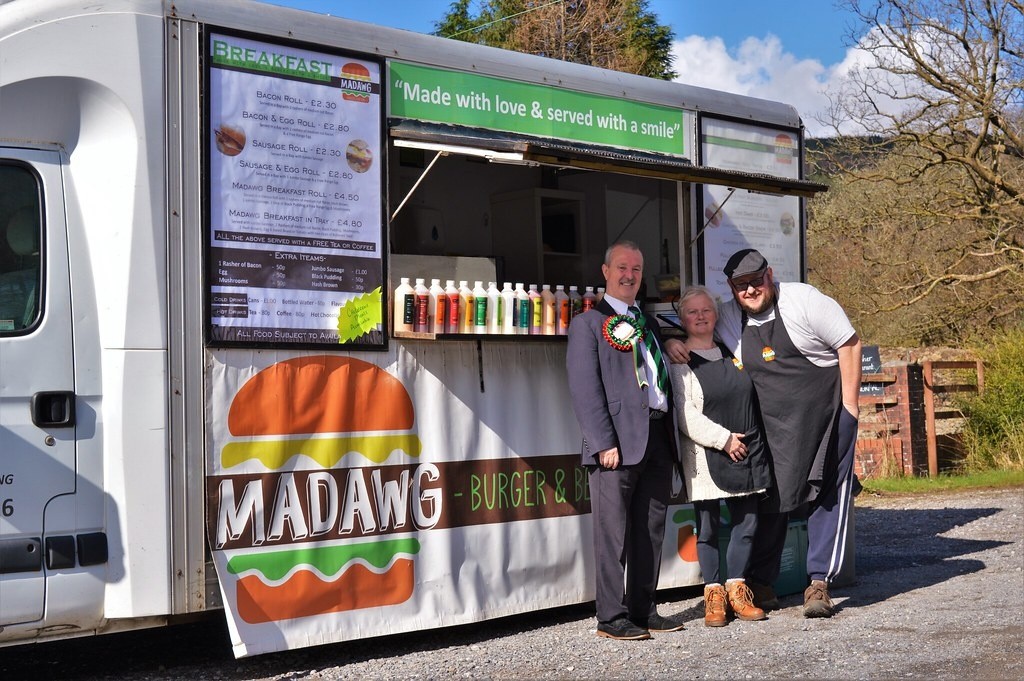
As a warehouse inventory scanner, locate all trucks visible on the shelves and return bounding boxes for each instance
[1,0,828,648]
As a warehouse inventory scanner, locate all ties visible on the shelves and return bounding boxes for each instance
[628,306,674,412]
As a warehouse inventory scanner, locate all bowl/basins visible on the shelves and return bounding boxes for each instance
[653,274,680,291]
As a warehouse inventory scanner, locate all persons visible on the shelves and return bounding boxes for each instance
[566,239,685,639]
[662,249,861,627]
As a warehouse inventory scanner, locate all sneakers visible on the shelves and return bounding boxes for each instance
[743,580,781,610]
[804,581,836,616]
[630,615,685,631]
[597,617,651,639]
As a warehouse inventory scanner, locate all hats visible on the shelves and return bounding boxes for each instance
[723,249,768,279]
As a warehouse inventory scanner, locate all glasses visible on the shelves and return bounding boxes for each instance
[735,269,768,290]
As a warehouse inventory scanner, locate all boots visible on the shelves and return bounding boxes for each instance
[725,582,767,620]
[704,586,727,626]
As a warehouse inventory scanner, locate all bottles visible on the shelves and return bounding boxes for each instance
[472,281,487,334]
[427,279,445,333]
[444,280,458,333]
[554,285,569,335]
[501,282,513,334]
[394,278,415,332]
[568,286,583,321]
[413,278,430,333]
[583,287,595,313]
[485,282,501,334]
[597,288,605,303]
[527,285,542,335]
[513,283,529,335]
[540,285,556,335]
[458,281,474,334]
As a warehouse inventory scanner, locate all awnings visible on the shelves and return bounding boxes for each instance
[390,125,830,201]
